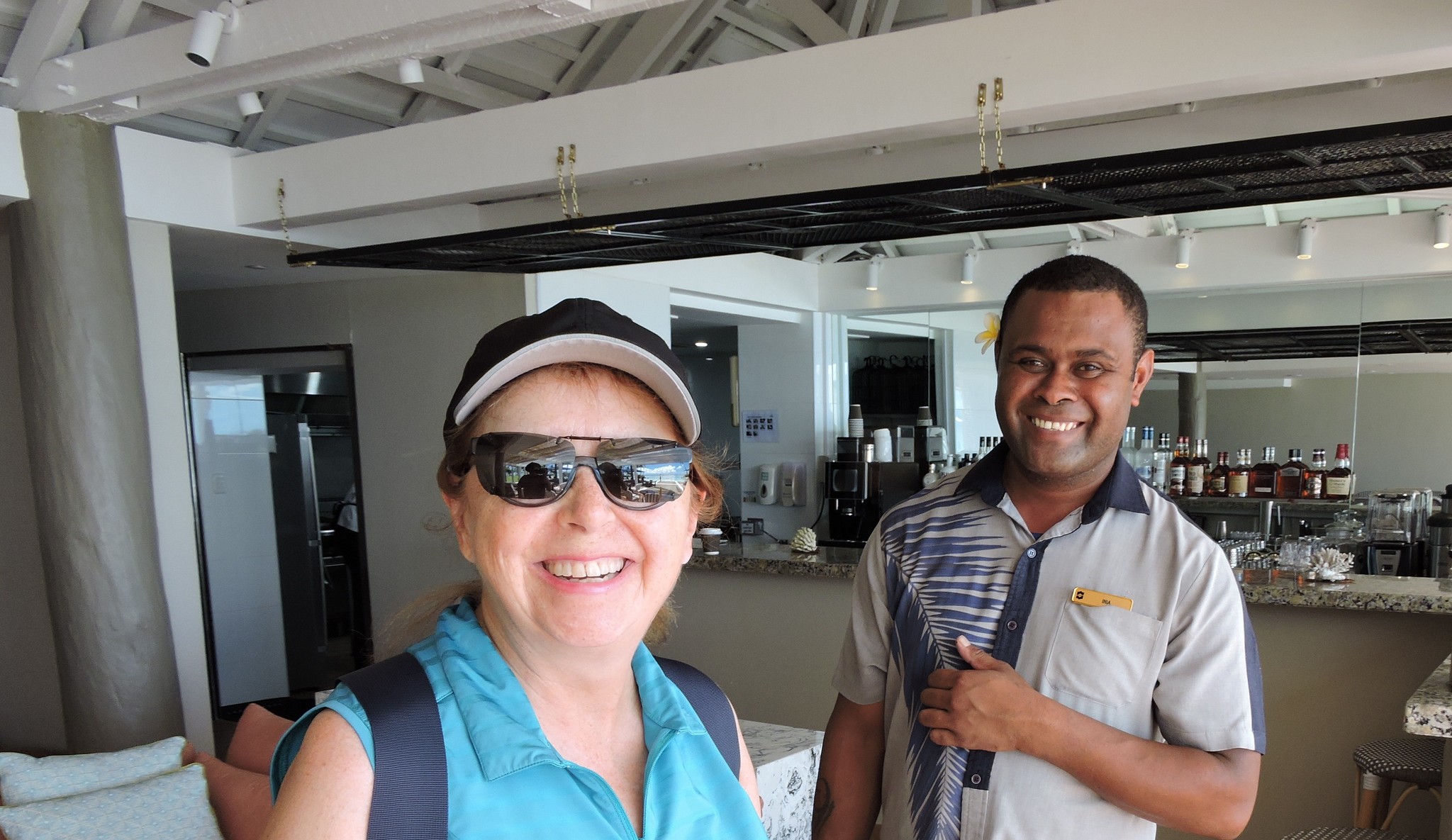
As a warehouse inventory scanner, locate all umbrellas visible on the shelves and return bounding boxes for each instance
[623,469,645,472]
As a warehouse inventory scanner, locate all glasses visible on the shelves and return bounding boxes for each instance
[451,432,693,508]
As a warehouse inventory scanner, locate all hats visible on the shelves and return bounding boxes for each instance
[444,298,700,475]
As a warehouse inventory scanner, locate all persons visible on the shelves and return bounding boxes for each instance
[812,253,1266,840]
[265,297,767,840]
[517,462,552,499]
[551,477,559,490]
[659,474,661,481]
[597,462,627,499]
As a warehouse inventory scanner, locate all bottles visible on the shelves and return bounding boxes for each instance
[923,436,1003,487]
[1318,512,1358,574]
[1428,484,1452,578]
[1343,509,1367,574]
[1119,426,1350,501]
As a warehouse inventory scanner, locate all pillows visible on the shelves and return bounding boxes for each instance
[0,701,301,840]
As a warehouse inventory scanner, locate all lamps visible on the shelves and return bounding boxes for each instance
[1432,204,1452,251]
[1293,216,1319,262]
[397,57,426,86]
[183,1,240,70]
[1173,229,1197,269]
[234,91,265,118]
[959,247,979,286]
[863,256,883,292]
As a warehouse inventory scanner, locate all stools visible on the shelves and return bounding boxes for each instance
[1352,736,1445,835]
[1279,826,1418,840]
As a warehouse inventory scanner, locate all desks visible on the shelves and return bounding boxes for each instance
[736,717,828,840]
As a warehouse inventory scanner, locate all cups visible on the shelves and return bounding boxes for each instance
[1229,532,1319,541]
[916,406,933,426]
[698,528,723,555]
[858,427,892,463]
[849,404,864,438]
[1217,539,1311,570]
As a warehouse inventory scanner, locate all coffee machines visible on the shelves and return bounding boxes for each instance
[817,461,920,549]
[1367,488,1419,577]
[1416,488,1433,576]
